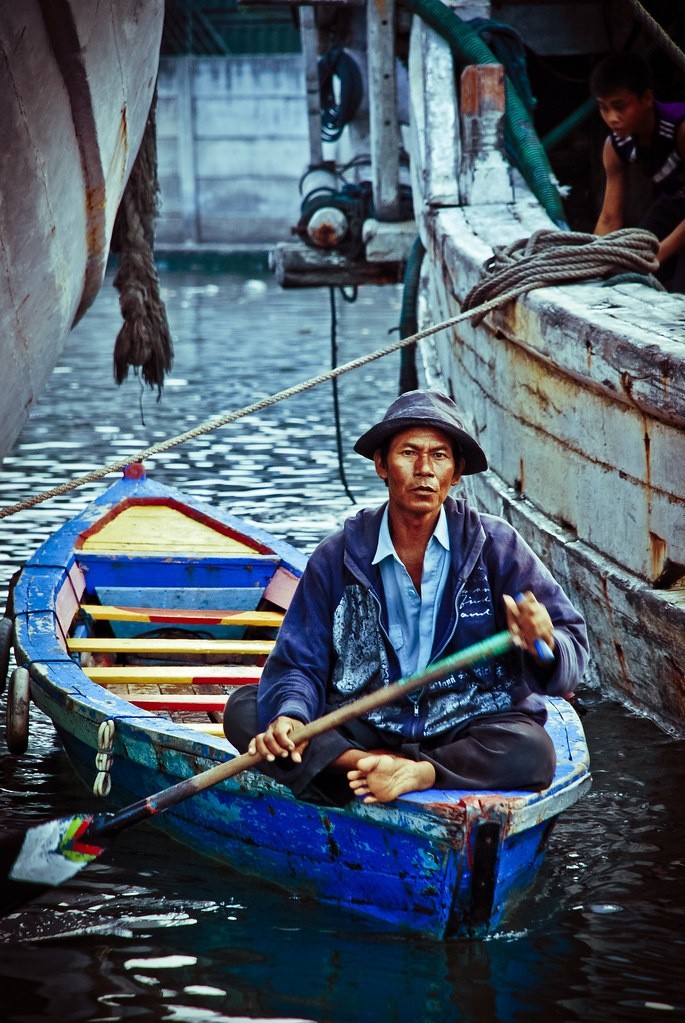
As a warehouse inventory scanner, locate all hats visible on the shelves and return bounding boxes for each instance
[352,390,489,476]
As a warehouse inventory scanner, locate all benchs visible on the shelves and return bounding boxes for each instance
[78,602,283,627]
[82,664,263,683]
[119,691,227,713]
[67,636,276,654]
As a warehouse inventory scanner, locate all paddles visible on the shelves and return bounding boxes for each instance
[0,589,559,910]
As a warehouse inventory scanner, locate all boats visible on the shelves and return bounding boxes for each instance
[0,459,595,951]
[265,0,685,746]
[0,0,166,464]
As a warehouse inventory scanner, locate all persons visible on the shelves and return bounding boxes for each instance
[591,56,685,294]
[224,389,590,809]
